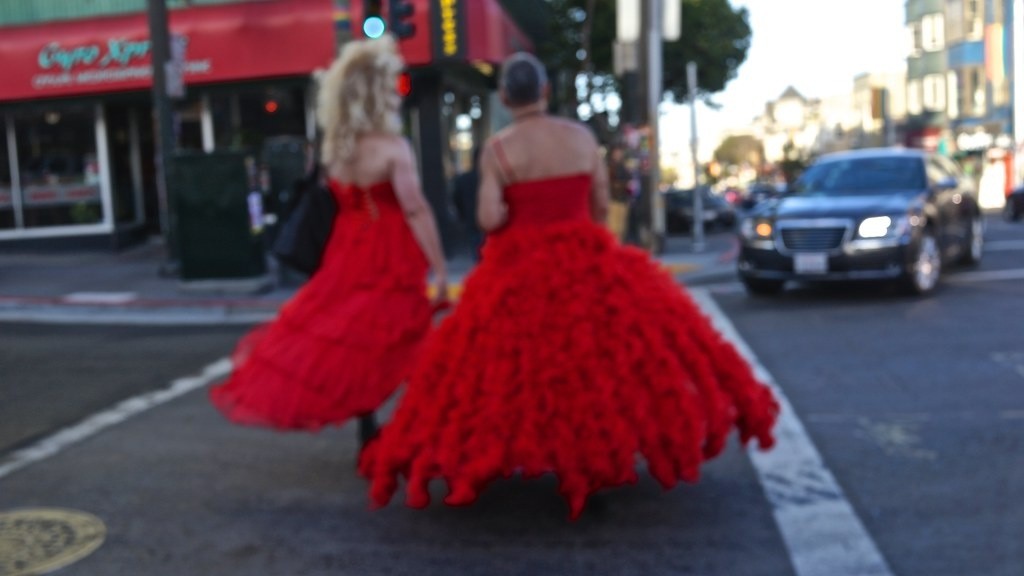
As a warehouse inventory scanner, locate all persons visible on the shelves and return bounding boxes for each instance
[318,41,448,445]
[474,52,610,490]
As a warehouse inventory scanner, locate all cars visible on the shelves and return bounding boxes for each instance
[735,146,985,298]
[669,182,732,234]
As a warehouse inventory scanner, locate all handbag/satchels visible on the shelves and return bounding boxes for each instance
[273,186,338,277]
